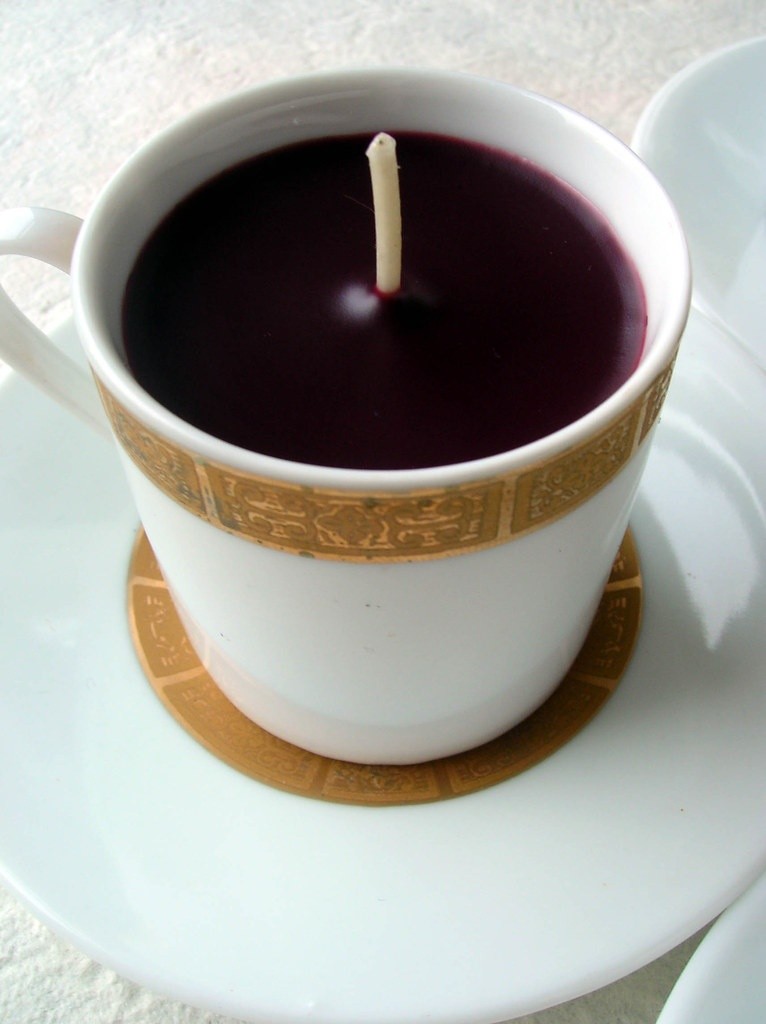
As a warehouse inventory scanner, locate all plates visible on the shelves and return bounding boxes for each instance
[632,40,766,353]
[0,303,766,1024]
[655,872,766,1024]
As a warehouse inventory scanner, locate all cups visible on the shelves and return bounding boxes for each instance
[0,71,692,767]
